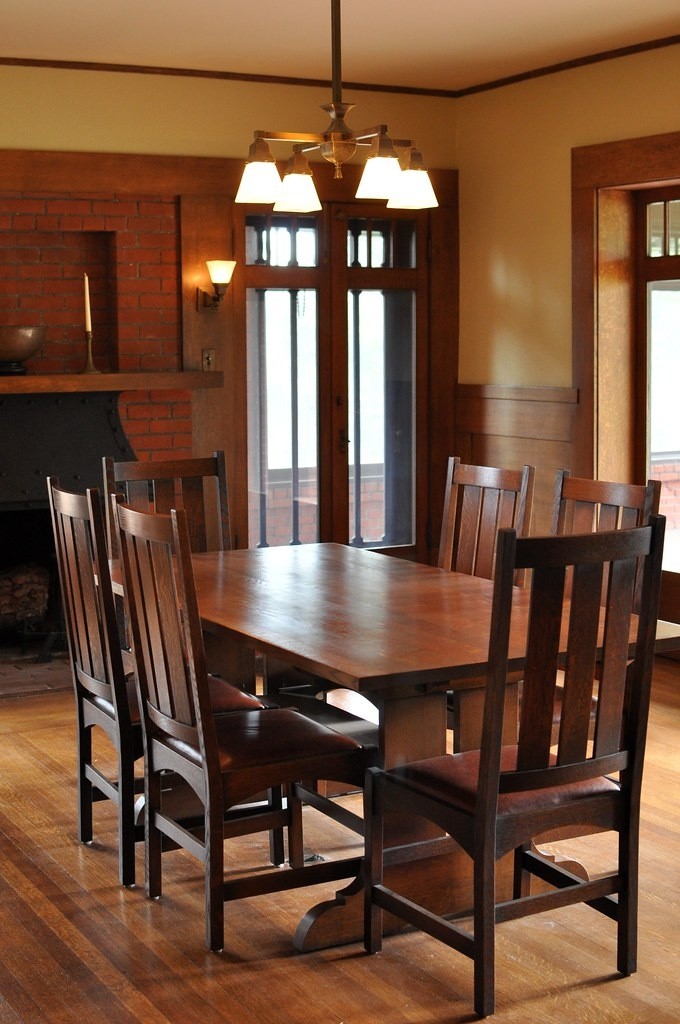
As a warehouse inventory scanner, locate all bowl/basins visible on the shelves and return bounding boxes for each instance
[0,326,47,376]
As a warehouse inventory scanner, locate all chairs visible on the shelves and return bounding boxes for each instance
[367,512,670,1017]
[110,493,368,952]
[436,468,662,750]
[100,451,234,680]
[46,474,287,900]
[437,456,536,589]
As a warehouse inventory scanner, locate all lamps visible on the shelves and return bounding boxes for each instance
[196,260,237,312]
[234,0,439,213]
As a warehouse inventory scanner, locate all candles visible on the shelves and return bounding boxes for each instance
[81,273,94,332]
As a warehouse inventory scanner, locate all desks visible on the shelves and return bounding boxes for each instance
[90,542,679,953]
